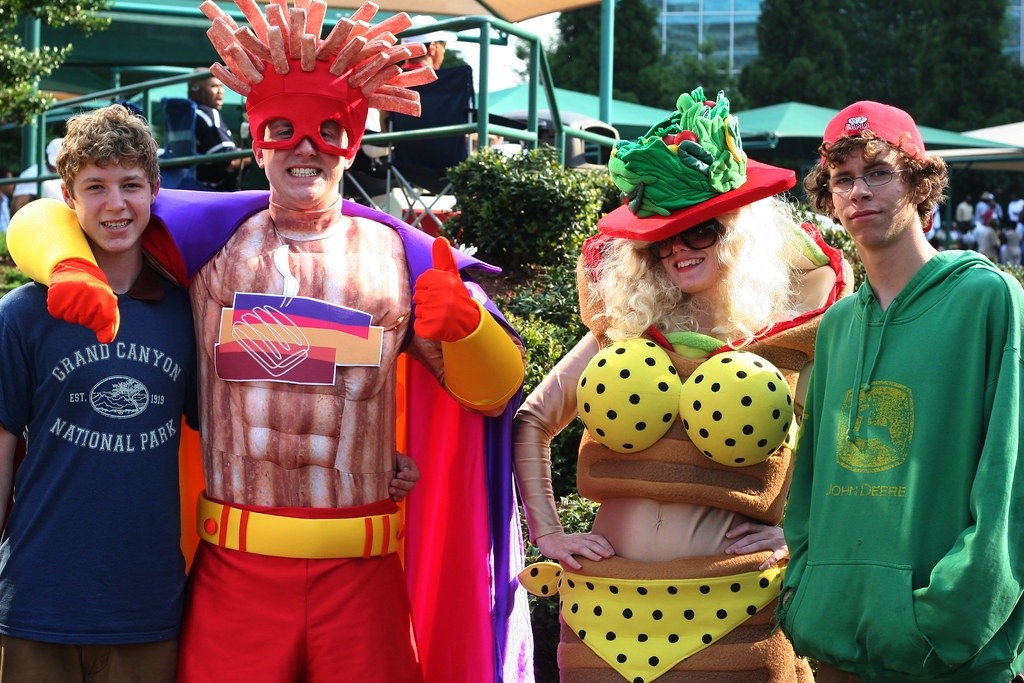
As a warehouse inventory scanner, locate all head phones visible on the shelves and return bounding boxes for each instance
[190,68,202,92]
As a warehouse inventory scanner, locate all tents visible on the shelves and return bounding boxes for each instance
[469,83,1024,251]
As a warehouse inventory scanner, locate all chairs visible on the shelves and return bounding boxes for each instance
[158,97,217,190]
[385,66,477,246]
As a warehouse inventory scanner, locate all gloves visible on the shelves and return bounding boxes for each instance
[46,257,120,343]
[411,236,481,344]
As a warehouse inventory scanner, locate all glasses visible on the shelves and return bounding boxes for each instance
[647,219,720,259]
[822,168,909,192]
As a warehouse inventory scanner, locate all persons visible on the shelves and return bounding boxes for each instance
[513,86,853,683]
[0,0,525,683]
[777,101,1024,683]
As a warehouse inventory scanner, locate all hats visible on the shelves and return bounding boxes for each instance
[597,85,797,243]
[820,99,926,170]
[401,15,457,45]
[197,0,438,160]
